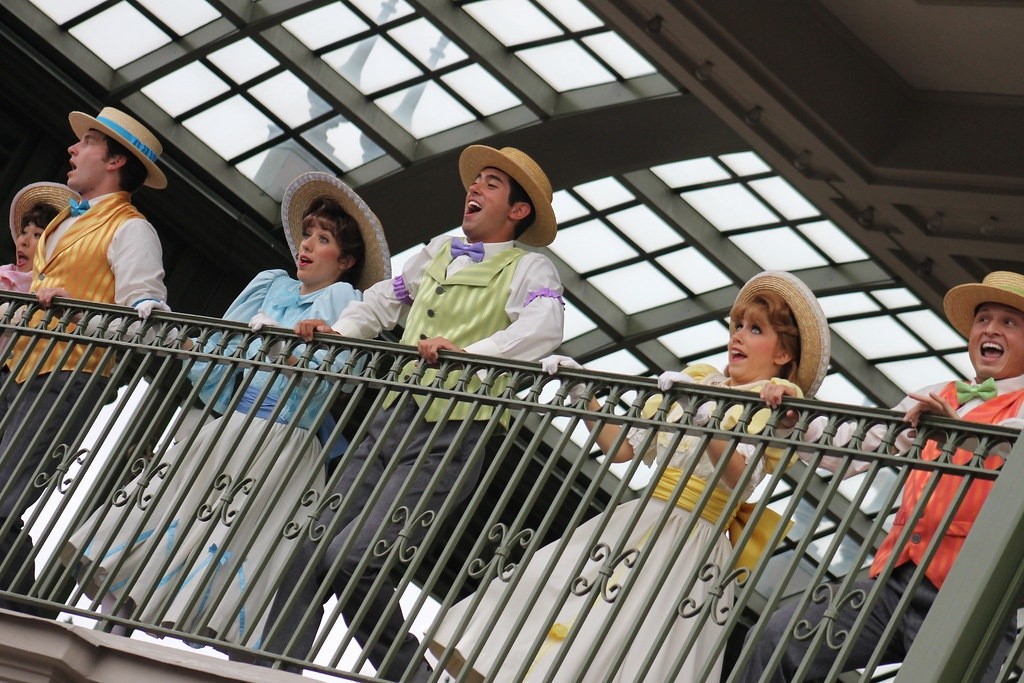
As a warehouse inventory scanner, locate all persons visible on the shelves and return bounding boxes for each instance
[252,143,562,683]
[66,169,377,651]
[741,270,1024,683]
[1,104,166,609]
[422,272,827,683]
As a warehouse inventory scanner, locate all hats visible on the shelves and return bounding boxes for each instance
[459,145,557,246]
[729,271,831,399]
[943,272,1024,342]
[10,181,82,245]
[69,106,168,189]
[280,171,392,292]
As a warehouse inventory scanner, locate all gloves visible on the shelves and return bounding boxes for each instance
[657,371,696,411]
[539,354,587,406]
[132,297,178,345]
[247,308,286,363]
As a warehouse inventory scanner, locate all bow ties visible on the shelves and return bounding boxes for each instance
[69,198,90,217]
[954,377,996,404]
[450,237,485,263]
